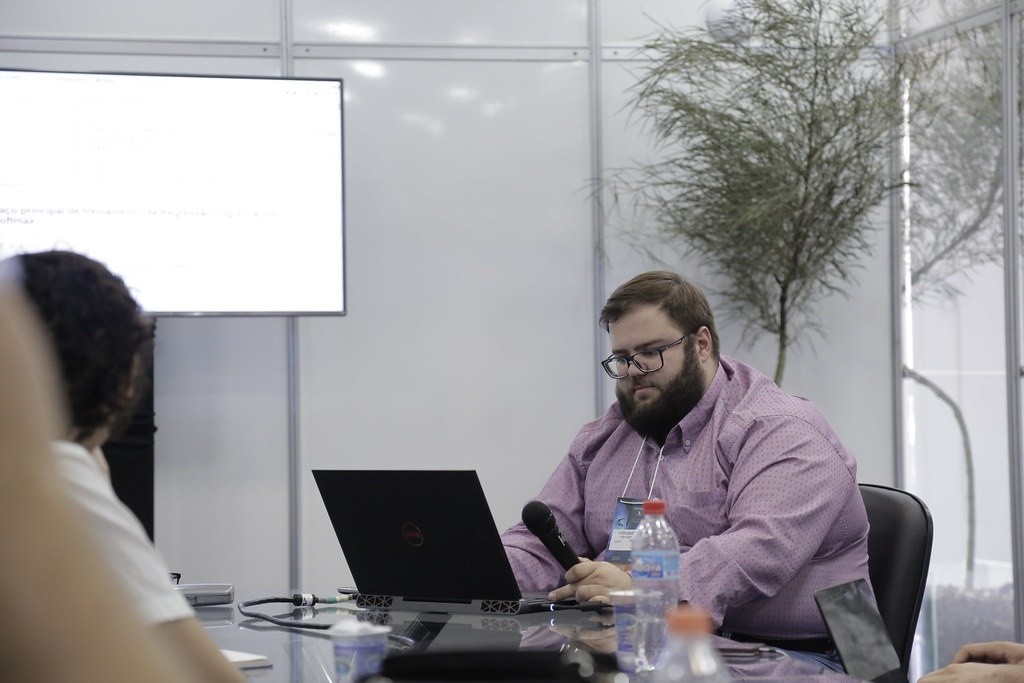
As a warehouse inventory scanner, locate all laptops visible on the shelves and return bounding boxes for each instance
[311,469,578,615]
[814,577,910,682]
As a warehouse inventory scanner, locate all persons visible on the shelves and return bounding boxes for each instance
[1,252,240,683]
[491,273,1024,683]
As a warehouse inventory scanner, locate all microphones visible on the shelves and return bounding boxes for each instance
[521,501,581,571]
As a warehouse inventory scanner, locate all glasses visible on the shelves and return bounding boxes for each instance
[600,335,691,379]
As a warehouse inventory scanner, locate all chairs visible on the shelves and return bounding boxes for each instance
[859,483,934,674]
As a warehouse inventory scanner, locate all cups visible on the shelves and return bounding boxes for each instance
[609,590,661,672]
[326,624,393,683]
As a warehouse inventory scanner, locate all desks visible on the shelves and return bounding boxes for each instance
[193,588,614,683]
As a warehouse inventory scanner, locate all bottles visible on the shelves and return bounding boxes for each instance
[630,501,679,673]
[654,610,731,683]
